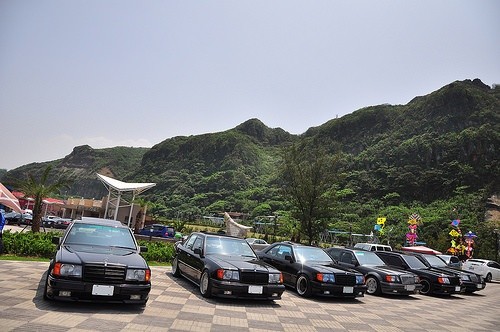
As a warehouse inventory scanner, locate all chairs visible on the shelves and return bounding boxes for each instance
[282,252,290,259]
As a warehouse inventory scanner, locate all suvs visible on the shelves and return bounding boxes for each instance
[354,243,392,252]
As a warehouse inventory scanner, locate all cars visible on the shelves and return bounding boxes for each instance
[437,254,464,267]
[39,215,73,230]
[170,231,286,300]
[463,258,500,283]
[43,216,151,307]
[410,252,486,296]
[4,211,33,226]
[325,247,423,296]
[242,238,271,252]
[255,240,367,300]
[372,250,471,296]
[140,223,176,238]
